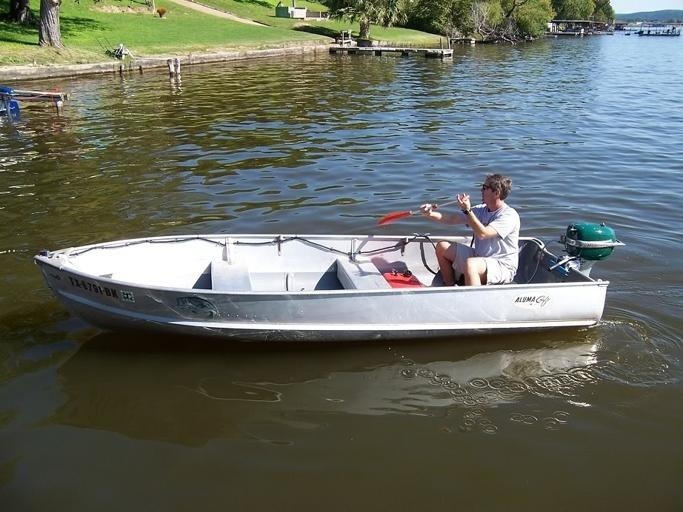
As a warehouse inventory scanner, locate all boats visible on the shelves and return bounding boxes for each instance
[50,334,599,449]
[33,222,625,343]
[639,27,680,36]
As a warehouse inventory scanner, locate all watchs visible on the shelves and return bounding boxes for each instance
[459,207,472,216]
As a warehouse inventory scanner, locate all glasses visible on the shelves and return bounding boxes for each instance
[482,184,489,191]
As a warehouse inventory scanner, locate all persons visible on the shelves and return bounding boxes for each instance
[418,173,520,287]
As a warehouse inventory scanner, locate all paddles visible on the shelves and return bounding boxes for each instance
[376,194,470,225]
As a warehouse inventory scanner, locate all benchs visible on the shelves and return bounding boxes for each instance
[211,261,251,291]
[337,255,390,288]
